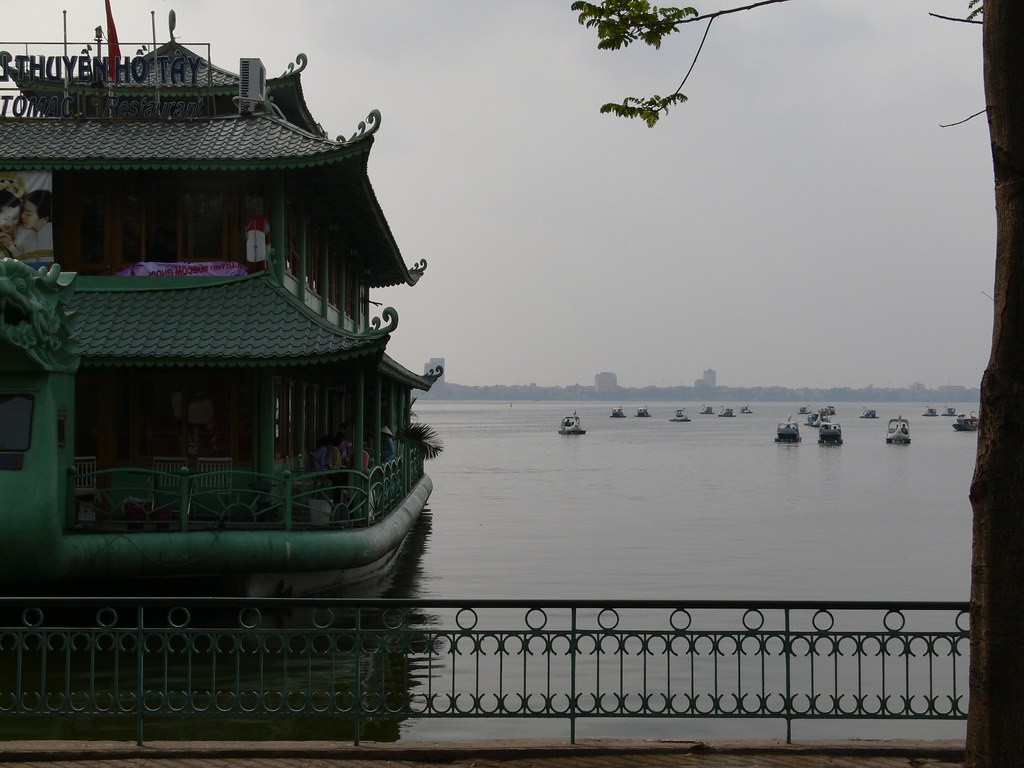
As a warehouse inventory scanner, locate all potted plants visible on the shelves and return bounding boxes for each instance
[308,480,334,526]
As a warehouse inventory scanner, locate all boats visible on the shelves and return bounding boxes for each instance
[886,413,912,445]
[803,404,837,426]
[739,405,753,414]
[699,403,714,414]
[922,405,939,416]
[634,404,652,417]
[558,409,587,435]
[718,405,737,418]
[610,404,627,419]
[669,407,692,422]
[817,413,843,445]
[952,410,979,432]
[859,405,879,418]
[777,413,800,440]
[940,406,958,417]
[797,402,813,415]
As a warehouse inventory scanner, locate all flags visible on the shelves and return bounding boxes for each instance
[106,0,121,80]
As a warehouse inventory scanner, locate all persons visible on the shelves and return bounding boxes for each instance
[562,403,980,447]
[0,188,55,271]
[309,419,395,525]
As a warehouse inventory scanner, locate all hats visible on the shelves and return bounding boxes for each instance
[381,425,395,436]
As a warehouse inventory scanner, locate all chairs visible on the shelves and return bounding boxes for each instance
[151,456,195,521]
[74,456,103,530]
[188,457,232,526]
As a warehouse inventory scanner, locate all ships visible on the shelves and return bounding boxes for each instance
[1,7,448,615]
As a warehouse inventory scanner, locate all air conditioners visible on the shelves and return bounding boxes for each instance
[238,58,265,113]
